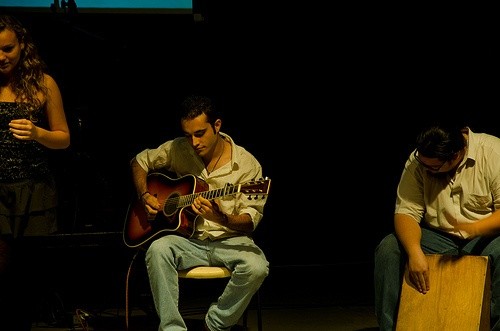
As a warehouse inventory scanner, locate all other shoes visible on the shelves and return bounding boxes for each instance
[71,305,92,331]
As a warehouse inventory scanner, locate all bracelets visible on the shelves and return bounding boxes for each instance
[138,191,149,203]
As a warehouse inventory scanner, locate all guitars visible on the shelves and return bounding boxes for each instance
[121,170,273,253]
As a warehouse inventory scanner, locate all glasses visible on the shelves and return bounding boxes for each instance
[414,149,446,171]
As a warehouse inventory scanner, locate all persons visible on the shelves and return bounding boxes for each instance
[0,17,70,331]
[373,118,500,331]
[131,97,270,331]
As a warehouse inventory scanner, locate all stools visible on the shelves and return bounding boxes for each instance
[177,266,263,331]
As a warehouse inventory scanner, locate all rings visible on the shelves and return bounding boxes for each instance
[198,207,203,210]
[146,212,149,215]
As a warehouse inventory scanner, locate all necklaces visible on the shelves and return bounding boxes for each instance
[206,141,225,176]
